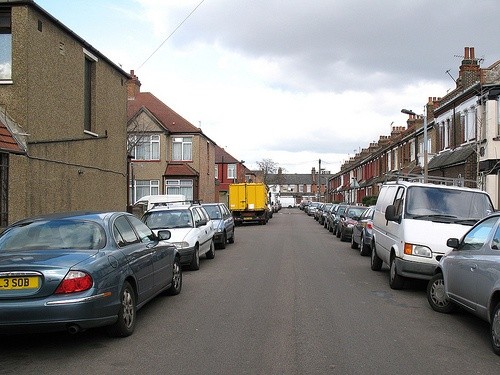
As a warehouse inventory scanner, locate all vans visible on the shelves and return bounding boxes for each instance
[370,174,496,293]
[130,194,188,220]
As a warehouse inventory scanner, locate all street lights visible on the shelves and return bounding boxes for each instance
[400,105,429,185]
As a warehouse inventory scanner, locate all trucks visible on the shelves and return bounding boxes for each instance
[228,182,272,225]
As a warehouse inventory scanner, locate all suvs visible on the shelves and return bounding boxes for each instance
[139,200,216,271]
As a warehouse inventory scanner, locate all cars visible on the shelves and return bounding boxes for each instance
[191,202,235,250]
[299,200,349,236]
[270,199,281,213]
[336,206,370,242]
[351,205,377,256]
[426,211,500,357]
[0,211,183,338]
